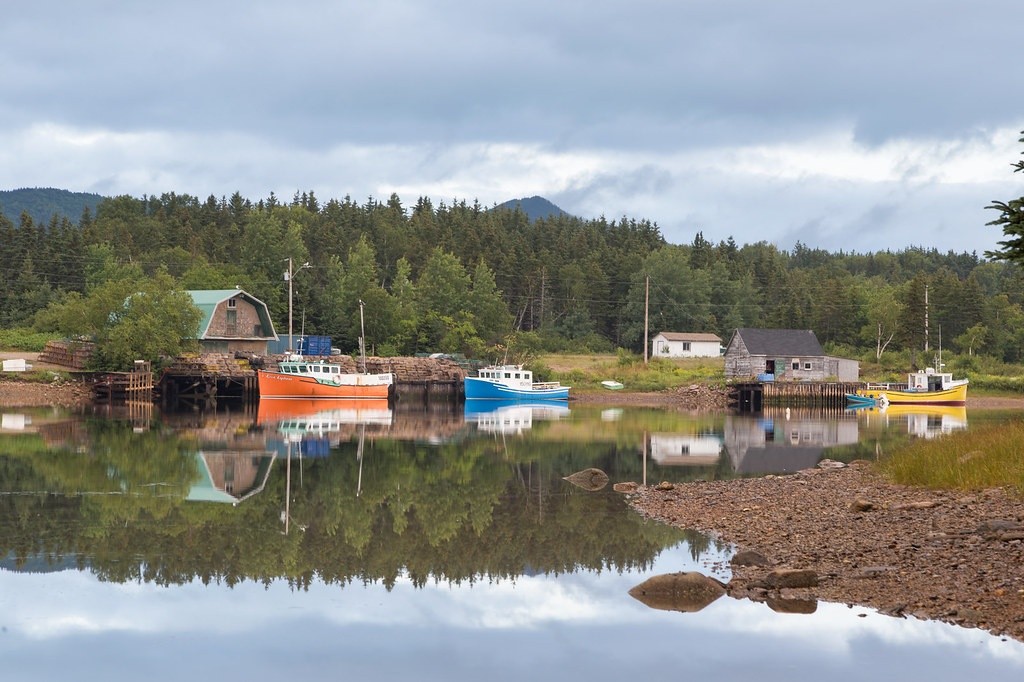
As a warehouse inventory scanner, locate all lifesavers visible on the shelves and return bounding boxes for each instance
[917,385,922,388]
[333,375,342,384]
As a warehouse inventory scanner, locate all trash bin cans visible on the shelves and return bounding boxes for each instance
[298,335,333,355]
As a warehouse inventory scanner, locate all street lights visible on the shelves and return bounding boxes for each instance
[288,264,312,354]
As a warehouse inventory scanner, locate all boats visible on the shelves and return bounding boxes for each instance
[855,404,970,442]
[844,402,889,414]
[256,348,395,399]
[858,322,970,406]
[601,380,624,391]
[463,398,572,459]
[846,393,890,405]
[464,362,573,400]
[256,396,394,536]
